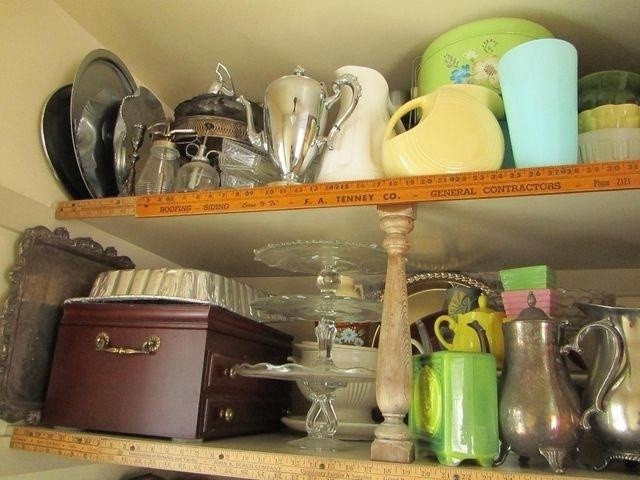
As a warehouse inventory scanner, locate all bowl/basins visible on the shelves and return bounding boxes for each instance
[294,340,380,423]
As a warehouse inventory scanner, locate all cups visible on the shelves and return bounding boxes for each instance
[577,70,640,113]
[578,104,640,134]
[501,288,561,317]
[499,264,558,291]
[579,127,640,164]
[499,39,579,170]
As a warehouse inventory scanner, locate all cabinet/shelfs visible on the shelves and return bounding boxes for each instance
[8,158,640,480]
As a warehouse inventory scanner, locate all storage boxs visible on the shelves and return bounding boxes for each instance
[38,304,293,442]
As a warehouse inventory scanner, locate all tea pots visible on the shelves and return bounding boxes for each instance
[236,65,362,184]
[434,292,518,369]
[571,302,639,474]
[466,290,626,474]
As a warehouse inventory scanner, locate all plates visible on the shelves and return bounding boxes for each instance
[112,86,166,192]
[372,286,447,359]
[409,308,446,355]
[62,267,280,324]
[281,416,377,441]
